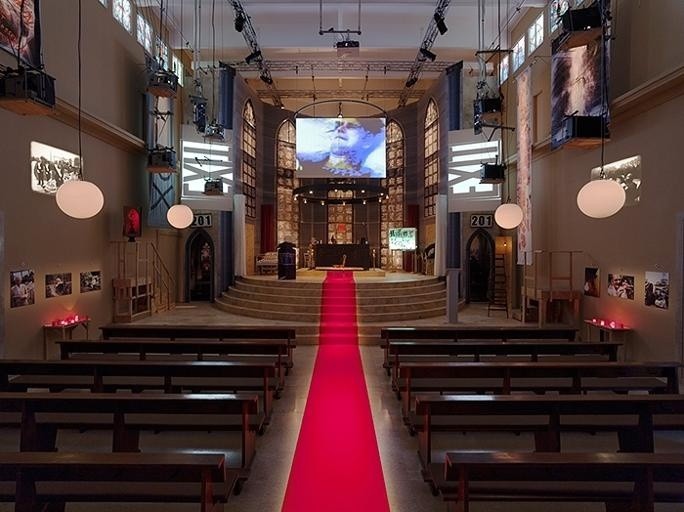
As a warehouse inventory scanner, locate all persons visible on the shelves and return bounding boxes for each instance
[608,278,633,299]
[11,277,30,306]
[26,272,35,303]
[295,117,386,179]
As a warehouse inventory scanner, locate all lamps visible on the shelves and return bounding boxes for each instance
[290,96,392,206]
[228,3,274,86]
[163,0,197,231]
[490,2,527,233]
[571,0,629,223]
[53,0,106,222]
[404,7,450,90]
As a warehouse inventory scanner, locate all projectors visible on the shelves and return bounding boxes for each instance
[336,40,359,60]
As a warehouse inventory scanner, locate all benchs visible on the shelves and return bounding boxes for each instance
[375,320,684,512]
[0,314,298,512]
